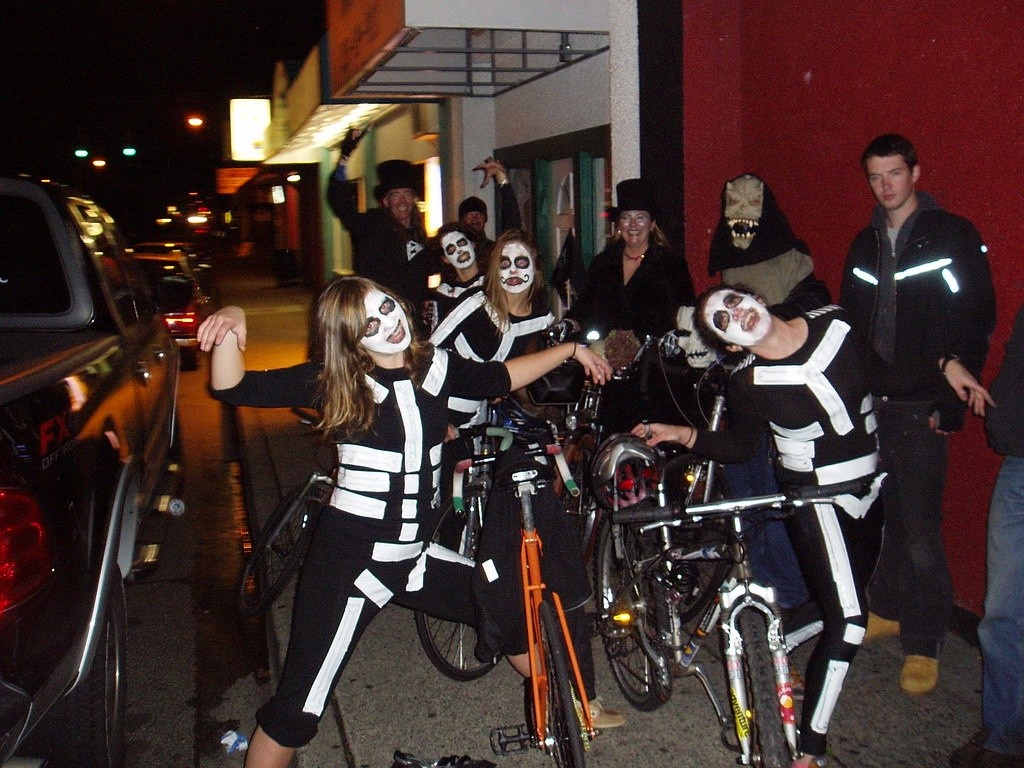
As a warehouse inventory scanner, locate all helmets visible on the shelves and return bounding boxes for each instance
[591,432,666,514]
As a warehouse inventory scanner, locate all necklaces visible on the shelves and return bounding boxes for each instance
[620,249,646,262]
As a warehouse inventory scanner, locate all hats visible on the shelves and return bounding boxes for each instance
[372,158,420,198]
[458,196,487,221]
[604,179,663,212]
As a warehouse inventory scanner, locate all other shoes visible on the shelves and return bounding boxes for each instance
[581,699,626,728]
[951,729,1020,768]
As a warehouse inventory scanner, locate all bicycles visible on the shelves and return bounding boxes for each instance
[231,330,870,768]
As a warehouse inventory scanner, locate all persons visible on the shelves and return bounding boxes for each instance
[834,132,998,695]
[950,296,1024,768]
[709,174,831,325]
[558,178,697,457]
[197,277,627,768]
[632,283,997,768]
[325,121,592,484]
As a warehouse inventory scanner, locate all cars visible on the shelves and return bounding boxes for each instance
[131,241,218,371]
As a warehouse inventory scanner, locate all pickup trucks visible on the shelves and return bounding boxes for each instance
[0,173,194,768]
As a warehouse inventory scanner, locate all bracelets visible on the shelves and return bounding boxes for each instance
[499,178,509,188]
[941,354,960,378]
[681,427,700,449]
[341,155,350,163]
[569,339,584,362]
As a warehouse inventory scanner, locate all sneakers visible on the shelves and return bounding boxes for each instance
[901,639,941,694]
[862,610,900,642]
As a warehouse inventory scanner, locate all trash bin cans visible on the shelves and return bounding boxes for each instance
[272,249,298,287]
